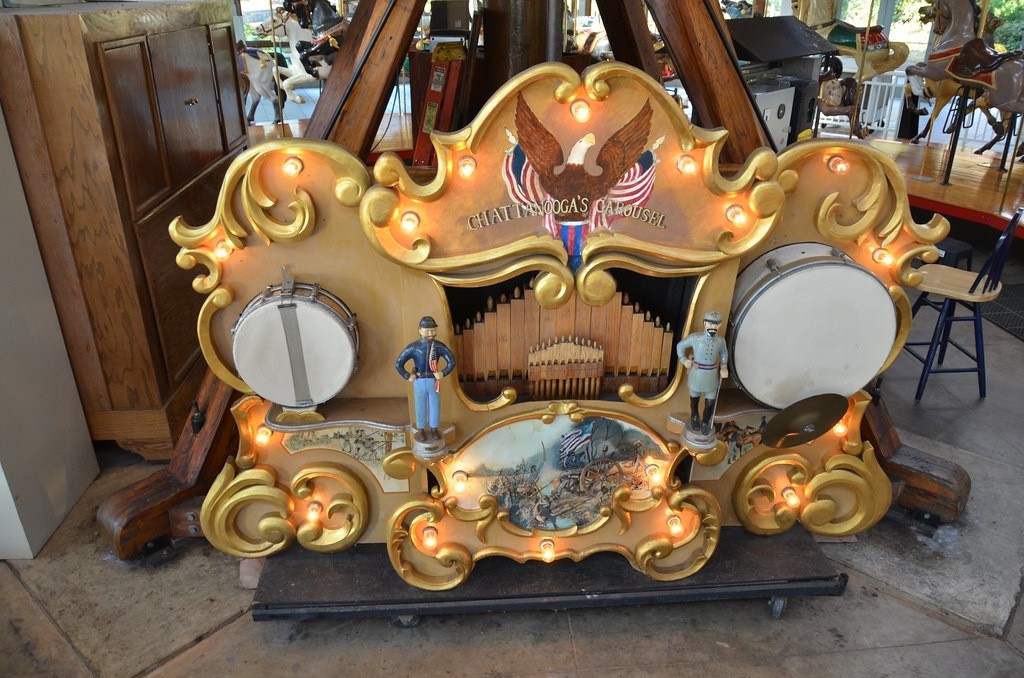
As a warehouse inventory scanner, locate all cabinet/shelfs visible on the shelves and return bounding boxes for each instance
[0,0,249,466]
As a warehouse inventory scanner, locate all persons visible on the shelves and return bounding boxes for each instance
[394,315,457,444]
[676,311,729,434]
[840,77,869,140]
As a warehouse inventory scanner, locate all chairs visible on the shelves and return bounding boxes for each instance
[876,207,1021,401]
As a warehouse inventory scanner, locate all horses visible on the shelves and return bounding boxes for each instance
[236,0,351,126]
[721,0,753,20]
[575,30,611,60]
[791,0,1024,158]
